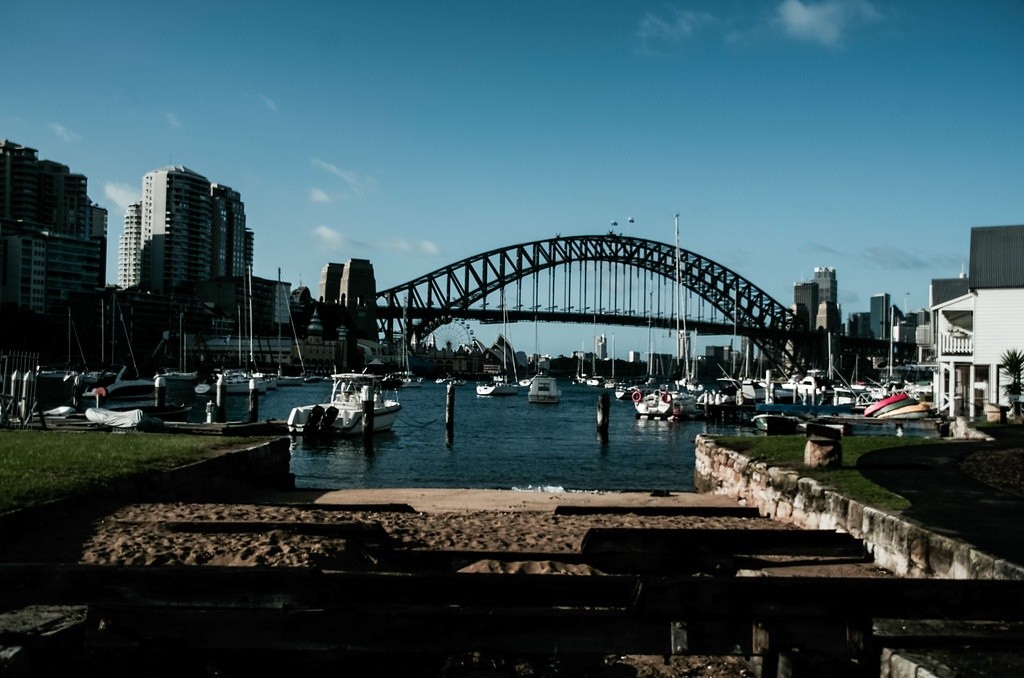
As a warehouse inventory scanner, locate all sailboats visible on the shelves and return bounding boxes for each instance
[276,267,305,387]
[37,307,89,379]
[152,313,199,386]
[83,299,119,383]
[393,297,422,387]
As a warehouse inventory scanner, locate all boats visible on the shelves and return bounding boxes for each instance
[322,377,333,382]
[528,374,562,403]
[571,213,933,436]
[435,373,454,384]
[32,380,193,425]
[475,290,520,396]
[212,369,267,395]
[299,372,323,383]
[288,373,403,437]
[228,265,278,391]
[453,380,467,386]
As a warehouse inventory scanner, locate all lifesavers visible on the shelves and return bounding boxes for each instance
[662,393,672,403]
[631,391,642,402]
